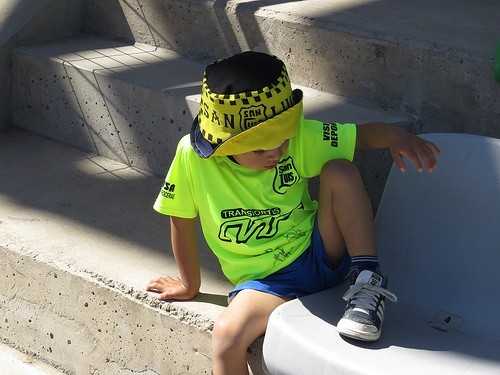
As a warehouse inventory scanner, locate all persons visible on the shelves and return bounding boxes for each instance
[147,51,443,375]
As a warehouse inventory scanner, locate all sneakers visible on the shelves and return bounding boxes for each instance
[336,265,398,343]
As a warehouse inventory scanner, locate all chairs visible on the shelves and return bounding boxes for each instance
[259,132,500,375]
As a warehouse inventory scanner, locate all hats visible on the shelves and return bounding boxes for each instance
[190,51,304,158]
